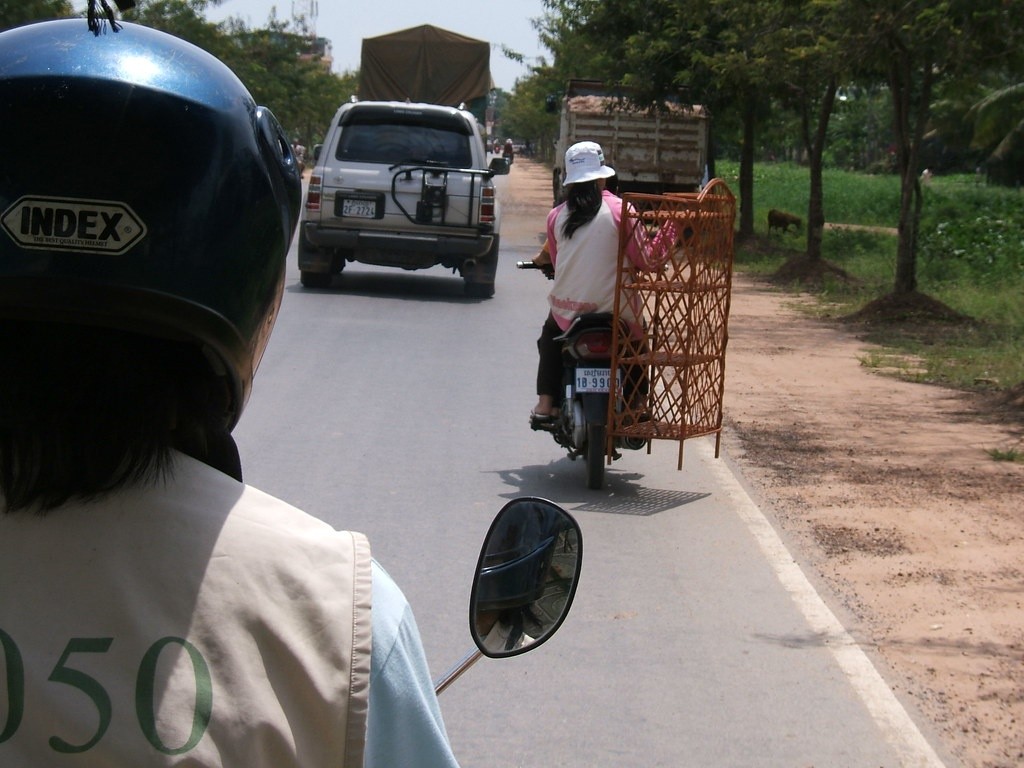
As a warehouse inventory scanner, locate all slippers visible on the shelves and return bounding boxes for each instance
[635,407,647,416]
[530,407,549,420]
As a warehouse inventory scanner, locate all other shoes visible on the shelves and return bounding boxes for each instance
[549,407,562,420]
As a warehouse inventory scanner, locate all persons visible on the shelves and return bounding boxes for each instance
[289,138,306,179]
[531,141,675,424]
[502,139,514,160]
[0,16,458,768]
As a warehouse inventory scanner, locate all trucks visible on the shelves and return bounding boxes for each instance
[545,77,708,247]
[360,23,492,152]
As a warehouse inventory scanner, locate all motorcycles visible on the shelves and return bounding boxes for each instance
[514,256,652,492]
[502,151,514,169]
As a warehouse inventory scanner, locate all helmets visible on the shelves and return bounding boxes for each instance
[0,20,302,435]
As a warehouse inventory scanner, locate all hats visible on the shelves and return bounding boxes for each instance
[506,139,512,144]
[563,141,615,186]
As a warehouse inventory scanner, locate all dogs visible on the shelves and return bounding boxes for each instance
[768,210,801,233]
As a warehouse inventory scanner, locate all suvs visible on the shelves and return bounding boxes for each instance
[298,95,511,300]
